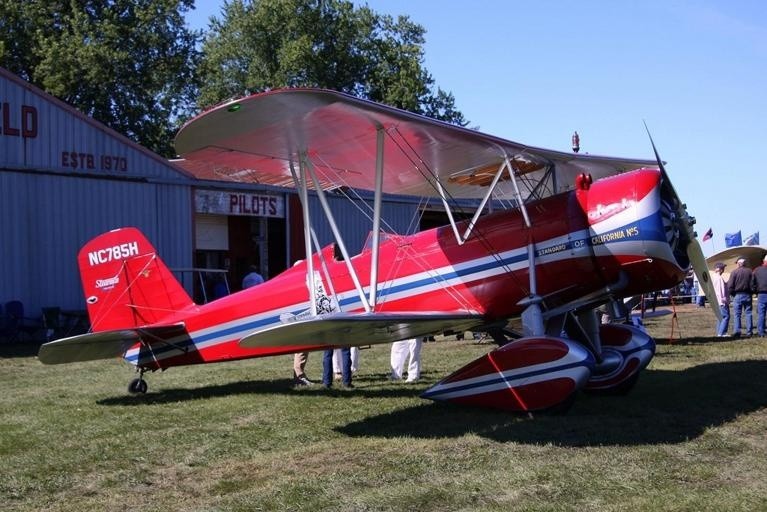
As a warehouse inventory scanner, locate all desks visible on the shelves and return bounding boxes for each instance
[60,309,89,337]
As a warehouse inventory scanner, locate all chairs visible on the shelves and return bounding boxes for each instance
[0,300,44,356]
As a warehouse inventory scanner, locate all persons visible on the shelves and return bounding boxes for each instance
[321,348,354,388]
[242,265,264,290]
[332,346,359,381]
[750,255,767,338]
[294,260,315,386]
[712,263,731,337]
[727,257,753,338]
[386,337,422,384]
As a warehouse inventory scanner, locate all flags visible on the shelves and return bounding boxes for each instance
[725,230,742,248]
[743,230,759,245]
[703,228,713,241]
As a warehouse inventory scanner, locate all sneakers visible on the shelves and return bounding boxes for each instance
[297,376,314,385]
[759,335,764,338]
[323,383,331,388]
[343,383,354,388]
[335,370,359,380]
[718,333,753,337]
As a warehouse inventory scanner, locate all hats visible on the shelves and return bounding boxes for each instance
[738,259,746,263]
[714,263,727,268]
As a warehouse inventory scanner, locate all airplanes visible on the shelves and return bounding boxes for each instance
[38,89,722,414]
